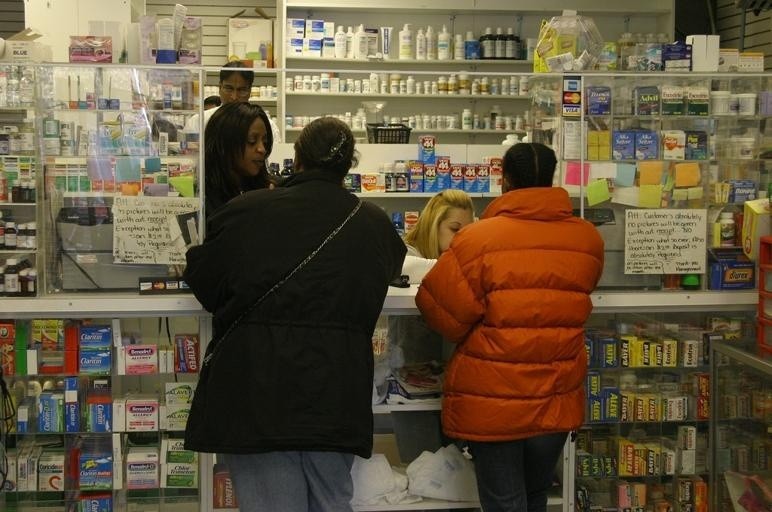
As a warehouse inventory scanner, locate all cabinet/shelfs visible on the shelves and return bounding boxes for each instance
[0,0,772,512]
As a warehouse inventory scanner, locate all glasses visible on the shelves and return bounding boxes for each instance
[219,84,251,97]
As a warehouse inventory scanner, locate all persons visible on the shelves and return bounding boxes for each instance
[415,143,605,512]
[183,60,281,144]
[203,96,221,111]
[194,102,282,217]
[389,189,474,464]
[183,117,408,512]
[152,121,178,142]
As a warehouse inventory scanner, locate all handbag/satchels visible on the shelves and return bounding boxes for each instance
[191,340,214,415]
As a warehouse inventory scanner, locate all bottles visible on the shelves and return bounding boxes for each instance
[437,23,453,60]
[481,27,494,59]
[415,83,423,94]
[347,79,354,93]
[514,31,522,58]
[5,221,16,250]
[383,162,397,192]
[356,24,368,59]
[734,138,756,159]
[335,25,346,58]
[432,82,438,94]
[398,24,416,60]
[505,118,512,130]
[389,114,457,129]
[294,75,303,91]
[448,76,459,94]
[494,28,505,60]
[19,119,35,156]
[346,26,355,59]
[6,178,35,203]
[326,108,369,130]
[472,79,481,95]
[286,78,294,90]
[407,75,416,94]
[311,76,321,93]
[380,27,394,59]
[437,76,448,94]
[730,93,739,114]
[416,29,426,59]
[424,81,432,94]
[391,81,399,94]
[710,90,731,113]
[369,71,378,94]
[26,222,36,250]
[501,79,510,95]
[465,32,482,60]
[266,85,272,97]
[0,254,36,296]
[516,118,523,130]
[462,109,472,129]
[474,113,479,129]
[426,25,437,59]
[519,76,528,96]
[320,74,330,93]
[399,80,407,93]
[491,78,498,95]
[380,80,388,93]
[510,76,518,95]
[523,111,530,131]
[0,213,5,249]
[490,104,502,129]
[0,170,8,201]
[739,94,757,116]
[396,164,409,191]
[458,70,470,94]
[0,131,11,155]
[454,34,466,59]
[303,76,312,92]
[480,76,490,94]
[719,212,735,247]
[506,28,517,59]
[259,85,267,97]
[16,224,27,249]
